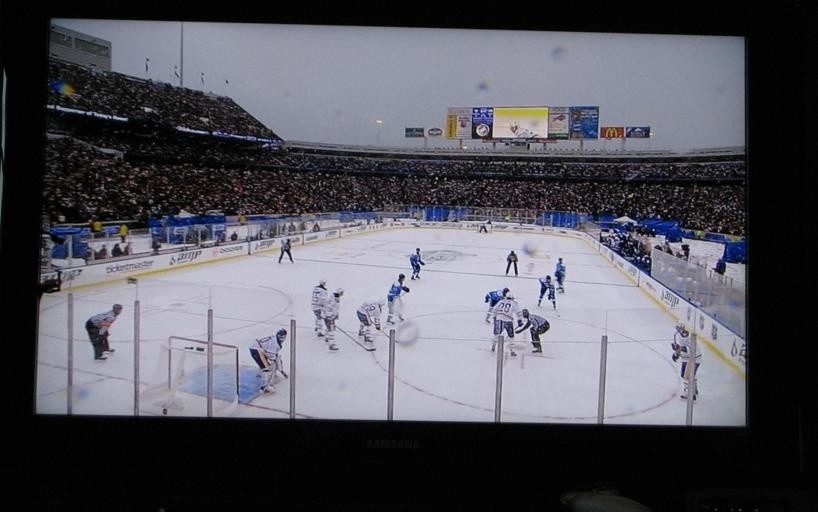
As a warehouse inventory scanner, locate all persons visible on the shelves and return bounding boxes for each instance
[484,287,510,323]
[323,287,344,352]
[250,329,287,396]
[538,275,559,309]
[386,273,409,327]
[356,299,386,349]
[40,58,747,282]
[670,320,704,402]
[311,275,330,339]
[491,291,524,356]
[516,306,551,354]
[85,303,122,360]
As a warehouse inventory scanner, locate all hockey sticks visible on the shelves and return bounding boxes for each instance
[334,325,376,352]
[547,295,561,317]
[256,338,289,379]
[371,321,405,343]
